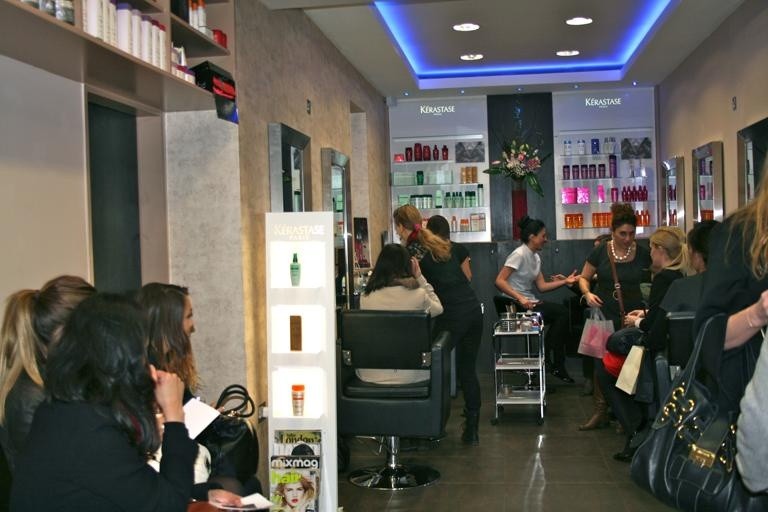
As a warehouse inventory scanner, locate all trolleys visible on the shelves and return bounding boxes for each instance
[491,311,549,426]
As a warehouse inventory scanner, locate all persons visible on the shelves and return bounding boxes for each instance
[274,471,314,512]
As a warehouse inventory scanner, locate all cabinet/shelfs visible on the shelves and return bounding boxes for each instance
[261,211,341,512]
[552,126,657,241]
[388,133,492,244]
[0,1,238,119]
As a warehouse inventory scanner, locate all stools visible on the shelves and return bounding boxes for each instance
[493,295,557,396]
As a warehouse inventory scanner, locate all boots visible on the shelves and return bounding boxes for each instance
[579,371,609,430]
[461,410,480,446]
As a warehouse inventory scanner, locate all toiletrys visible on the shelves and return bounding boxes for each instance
[289,315,303,352]
[393,142,486,233]
[668,185,677,227]
[700,184,705,199]
[289,252,301,285]
[290,383,305,417]
[701,158,705,174]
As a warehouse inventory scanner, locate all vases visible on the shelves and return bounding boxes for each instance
[510,178,528,242]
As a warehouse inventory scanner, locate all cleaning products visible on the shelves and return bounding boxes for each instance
[560,136,650,228]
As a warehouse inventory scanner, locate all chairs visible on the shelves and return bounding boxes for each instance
[650,310,696,410]
[337,309,459,491]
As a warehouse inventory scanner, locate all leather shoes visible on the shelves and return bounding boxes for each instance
[613,438,633,460]
[551,364,576,384]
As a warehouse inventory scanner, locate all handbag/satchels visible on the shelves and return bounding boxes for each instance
[615,333,655,403]
[577,306,615,359]
[629,312,768,512]
[195,384,259,478]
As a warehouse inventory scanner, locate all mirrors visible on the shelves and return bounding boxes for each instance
[266,118,313,219]
[351,213,373,271]
[735,116,767,208]
[688,142,727,226]
[320,146,354,309]
[659,153,687,232]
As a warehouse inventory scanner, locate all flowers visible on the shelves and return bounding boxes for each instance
[478,139,551,196]
